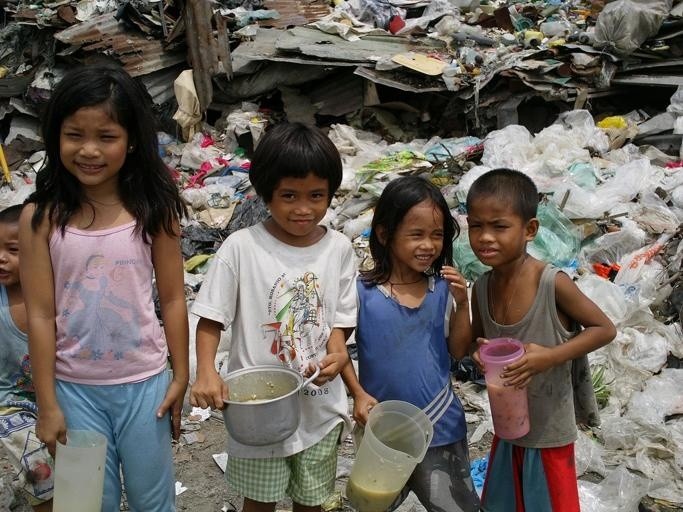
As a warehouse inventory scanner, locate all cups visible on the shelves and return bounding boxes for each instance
[479,337,531,440]
[52,429,108,512]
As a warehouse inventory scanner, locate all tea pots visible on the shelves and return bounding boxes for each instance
[343,399,434,512]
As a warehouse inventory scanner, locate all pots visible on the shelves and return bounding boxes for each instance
[219,360,321,445]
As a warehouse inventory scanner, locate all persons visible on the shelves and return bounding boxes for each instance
[336,174,482,512]
[0,204,55,512]
[462,167,617,512]
[16,64,191,512]
[190,120,359,512]
